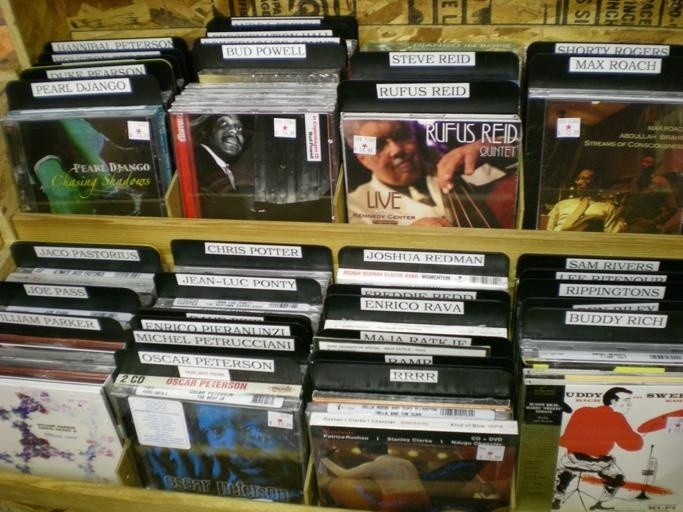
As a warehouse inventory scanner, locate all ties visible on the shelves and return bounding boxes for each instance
[561,198,590,230]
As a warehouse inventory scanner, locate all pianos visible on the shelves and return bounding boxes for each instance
[416,460,508,512]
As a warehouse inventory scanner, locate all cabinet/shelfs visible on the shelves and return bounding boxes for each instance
[0,0,683,512]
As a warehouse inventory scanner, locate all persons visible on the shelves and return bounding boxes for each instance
[19,118,143,215]
[341,118,520,226]
[147,400,303,503]
[318,440,490,511]
[547,167,623,233]
[620,154,678,232]
[194,114,244,194]
